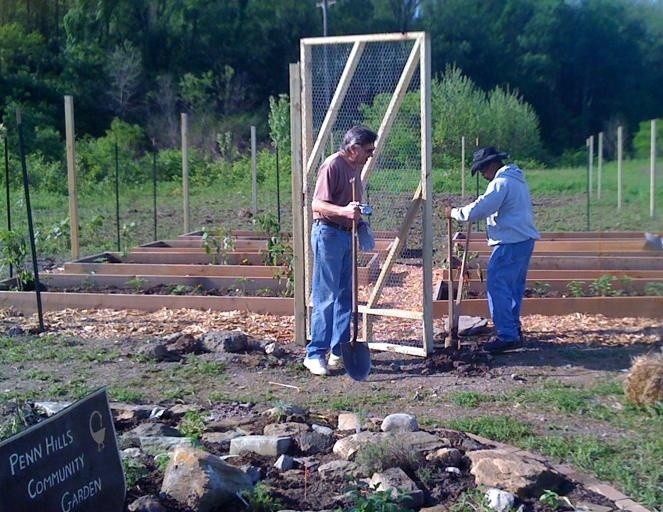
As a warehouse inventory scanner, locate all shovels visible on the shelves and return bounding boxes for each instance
[339,177,371,382]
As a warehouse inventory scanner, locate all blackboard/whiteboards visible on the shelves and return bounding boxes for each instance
[1,386,131,511]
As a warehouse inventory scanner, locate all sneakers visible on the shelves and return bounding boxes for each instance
[302,353,330,375]
[326,352,344,370]
[483,338,521,351]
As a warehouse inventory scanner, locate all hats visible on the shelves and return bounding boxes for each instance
[471,146,507,176]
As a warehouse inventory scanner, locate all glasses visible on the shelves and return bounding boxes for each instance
[480,168,487,176]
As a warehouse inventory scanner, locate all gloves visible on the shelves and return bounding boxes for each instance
[357,222,374,251]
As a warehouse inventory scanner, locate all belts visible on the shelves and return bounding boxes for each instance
[314,219,347,231]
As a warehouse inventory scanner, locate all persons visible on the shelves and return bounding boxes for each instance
[445,146,540,349]
[301,124,377,376]
